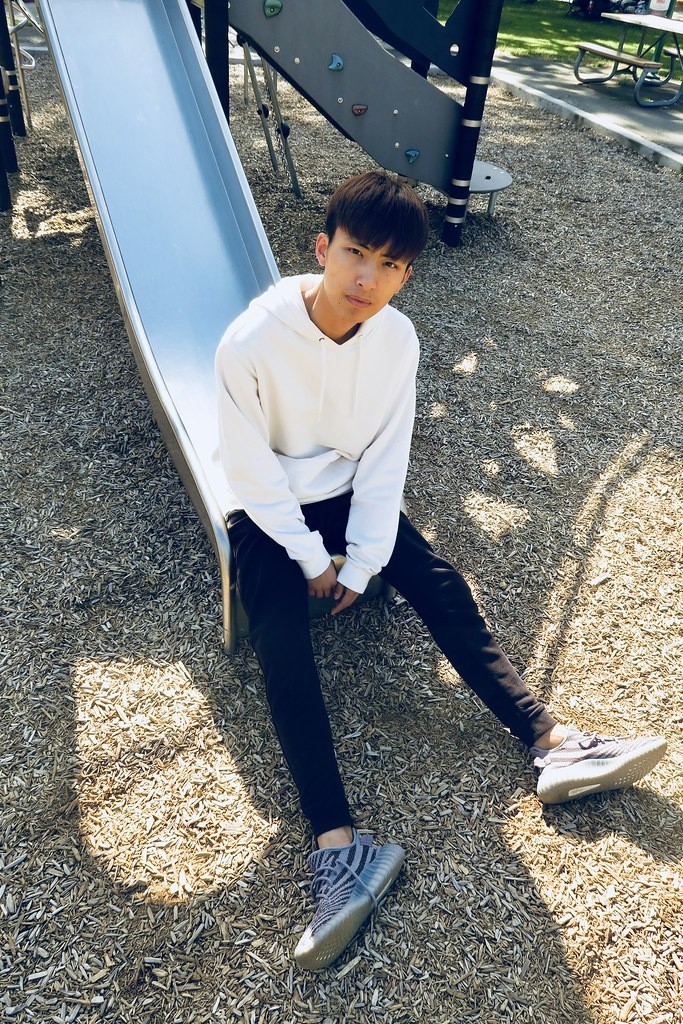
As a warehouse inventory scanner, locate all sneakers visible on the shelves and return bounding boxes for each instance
[293,828,406,969]
[530,727,667,808]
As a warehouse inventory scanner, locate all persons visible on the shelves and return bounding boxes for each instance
[214,171,668,971]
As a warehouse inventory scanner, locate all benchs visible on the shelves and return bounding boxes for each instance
[574,43,663,69]
[662,47,683,58]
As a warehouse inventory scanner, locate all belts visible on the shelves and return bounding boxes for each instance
[224,511,249,530]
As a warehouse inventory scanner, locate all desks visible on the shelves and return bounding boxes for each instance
[574,12,683,107]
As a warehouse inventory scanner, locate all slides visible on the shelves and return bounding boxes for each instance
[33,5,408,656]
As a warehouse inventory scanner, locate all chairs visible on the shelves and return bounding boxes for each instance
[565,0,591,20]
